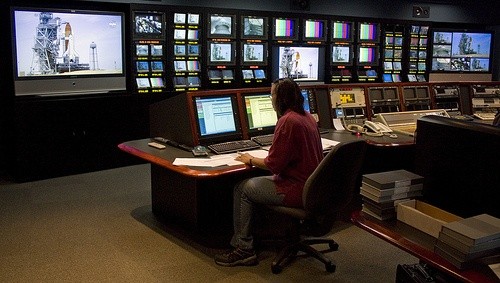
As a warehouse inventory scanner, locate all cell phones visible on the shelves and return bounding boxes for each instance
[192,149,208,157]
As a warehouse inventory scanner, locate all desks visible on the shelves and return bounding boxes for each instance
[116,127,362,246]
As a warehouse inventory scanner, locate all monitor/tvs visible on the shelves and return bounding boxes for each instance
[11,8,128,97]
[132,11,494,151]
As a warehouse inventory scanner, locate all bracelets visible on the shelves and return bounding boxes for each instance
[249,157,255,166]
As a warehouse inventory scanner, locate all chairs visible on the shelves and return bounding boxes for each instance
[255,139,364,273]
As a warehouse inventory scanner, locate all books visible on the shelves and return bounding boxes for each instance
[431,213,500,271]
[359,170,424,223]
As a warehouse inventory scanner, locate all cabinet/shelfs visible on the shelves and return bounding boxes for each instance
[349,118,500,283]
[0,92,147,185]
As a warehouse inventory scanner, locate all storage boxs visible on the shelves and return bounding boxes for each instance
[398,200,465,239]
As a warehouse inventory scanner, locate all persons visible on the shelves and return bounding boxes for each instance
[214,77,324,265]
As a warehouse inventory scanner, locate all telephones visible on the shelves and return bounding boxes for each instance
[363,120,393,134]
[348,124,363,133]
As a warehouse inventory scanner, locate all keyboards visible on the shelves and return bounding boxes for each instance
[208,140,261,155]
[251,134,275,146]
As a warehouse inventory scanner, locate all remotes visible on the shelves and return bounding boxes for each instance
[148,142,166,148]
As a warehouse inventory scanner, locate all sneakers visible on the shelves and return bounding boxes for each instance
[214,245,257,267]
[254,214,272,228]
